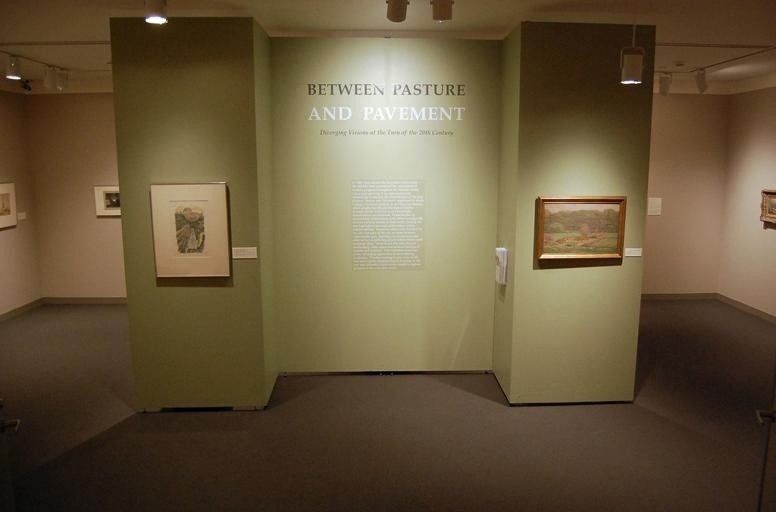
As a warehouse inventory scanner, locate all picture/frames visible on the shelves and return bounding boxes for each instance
[534,196,628,264]
[1,182,19,232]
[91,185,123,218]
[149,181,235,281]
[760,187,776,224]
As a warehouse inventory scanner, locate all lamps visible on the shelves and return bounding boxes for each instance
[382,1,412,23]
[142,0,172,25]
[617,0,651,84]
[428,0,457,24]
[1,52,71,93]
[654,68,711,100]
